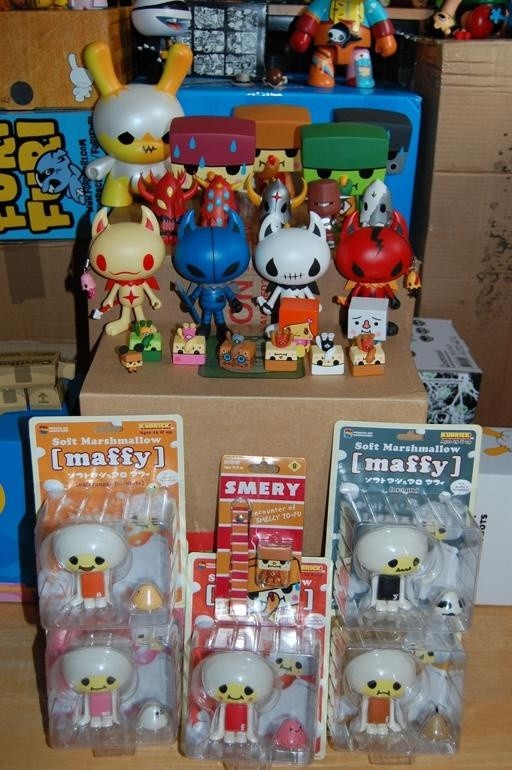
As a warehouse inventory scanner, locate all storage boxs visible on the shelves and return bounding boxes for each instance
[0,0,512,608]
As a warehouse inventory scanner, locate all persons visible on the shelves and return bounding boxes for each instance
[290,0,397,90]
